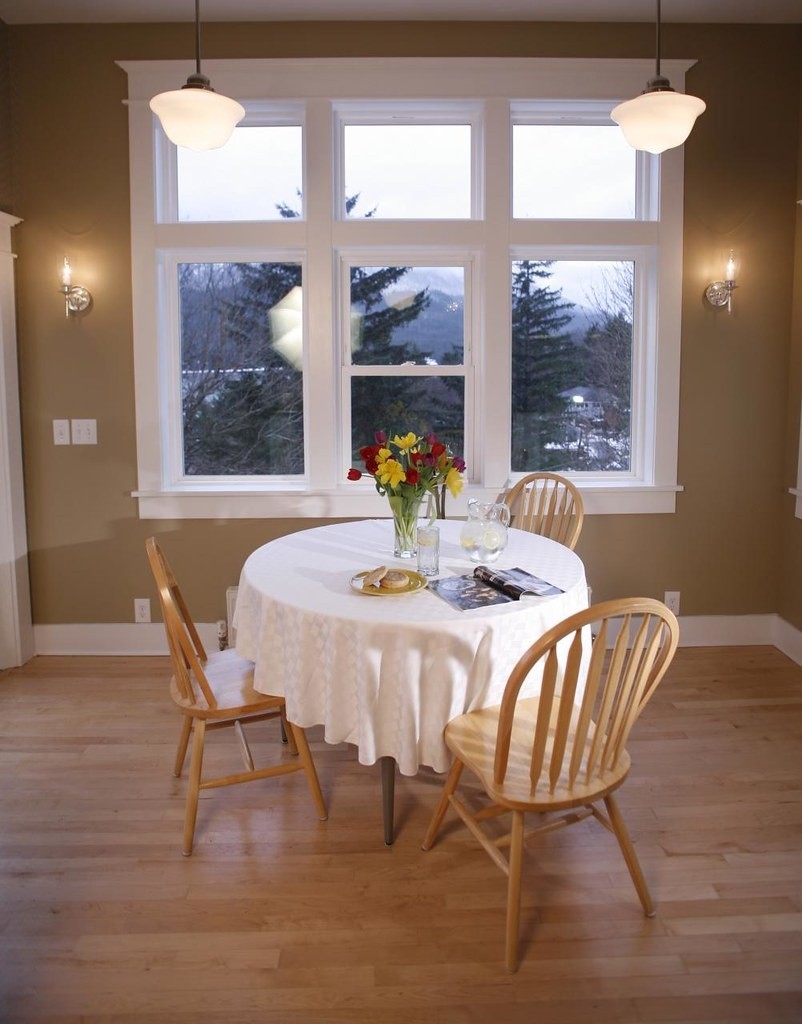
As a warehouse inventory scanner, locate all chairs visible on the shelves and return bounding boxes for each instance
[422,597,680,975]
[146,536,329,858]
[501,472,585,552]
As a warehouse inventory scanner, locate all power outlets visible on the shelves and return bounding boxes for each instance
[134,598,152,623]
[664,590,680,617]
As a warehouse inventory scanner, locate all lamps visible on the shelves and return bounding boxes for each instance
[705,248,737,315]
[149,0,246,149]
[58,256,92,319]
[610,1,706,155]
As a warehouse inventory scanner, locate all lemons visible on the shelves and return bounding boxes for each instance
[483,530,499,550]
[417,538,432,545]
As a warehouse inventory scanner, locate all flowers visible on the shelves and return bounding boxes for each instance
[345,428,467,555]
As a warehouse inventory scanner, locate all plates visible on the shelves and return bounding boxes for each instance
[349,568,429,596]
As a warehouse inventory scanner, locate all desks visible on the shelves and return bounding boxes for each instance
[231,518,592,847]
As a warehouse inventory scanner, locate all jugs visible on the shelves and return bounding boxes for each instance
[460,498,511,563]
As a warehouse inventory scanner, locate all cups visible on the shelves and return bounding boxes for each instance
[417,525,440,576]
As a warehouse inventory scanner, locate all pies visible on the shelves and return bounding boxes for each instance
[363,565,387,587]
[380,571,409,588]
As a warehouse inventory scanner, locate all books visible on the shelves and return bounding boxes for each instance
[426,566,568,614]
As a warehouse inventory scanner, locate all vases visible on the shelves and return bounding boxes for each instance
[386,484,425,558]
